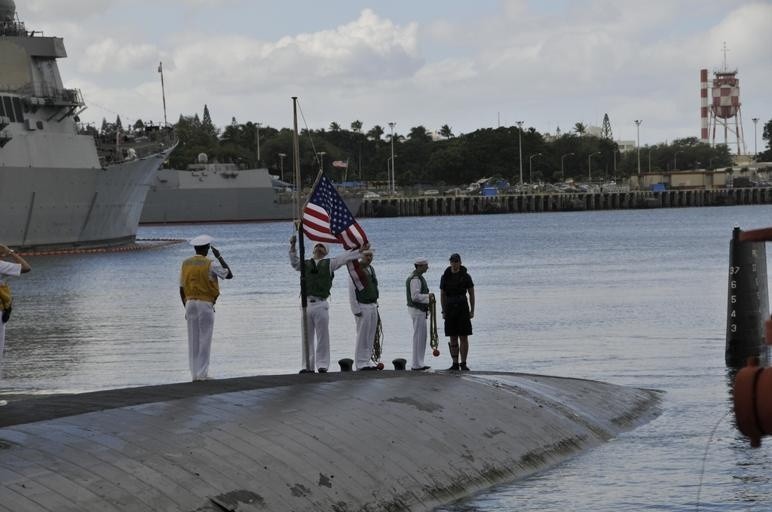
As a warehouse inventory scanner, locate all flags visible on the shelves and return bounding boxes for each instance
[302,174,368,293]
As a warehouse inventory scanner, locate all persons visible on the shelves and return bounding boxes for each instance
[439,253,475,372]
[179,234,233,381]
[405,256,434,372]
[0,245,32,407]
[349,249,380,371]
[288,235,369,374]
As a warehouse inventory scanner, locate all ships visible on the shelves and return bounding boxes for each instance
[139,153,364,224]
[0,0,180,255]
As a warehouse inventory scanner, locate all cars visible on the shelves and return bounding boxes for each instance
[423,190,440,196]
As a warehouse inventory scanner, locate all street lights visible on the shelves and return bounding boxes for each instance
[648,146,664,172]
[387,155,399,186]
[515,120,529,186]
[253,121,263,161]
[277,152,287,179]
[561,152,575,179]
[611,150,620,171]
[316,150,327,170]
[588,152,602,180]
[751,117,761,155]
[389,122,399,195]
[528,151,542,182]
[674,149,684,169]
[635,119,643,174]
[157,61,167,127]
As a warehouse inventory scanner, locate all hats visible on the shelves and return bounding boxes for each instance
[449,253,460,260]
[190,234,212,247]
[415,256,429,266]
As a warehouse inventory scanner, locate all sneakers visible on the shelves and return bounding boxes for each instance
[460,362,469,370]
[449,362,459,369]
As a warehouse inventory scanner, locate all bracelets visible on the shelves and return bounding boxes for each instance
[9,249,14,255]
[218,255,221,259]
